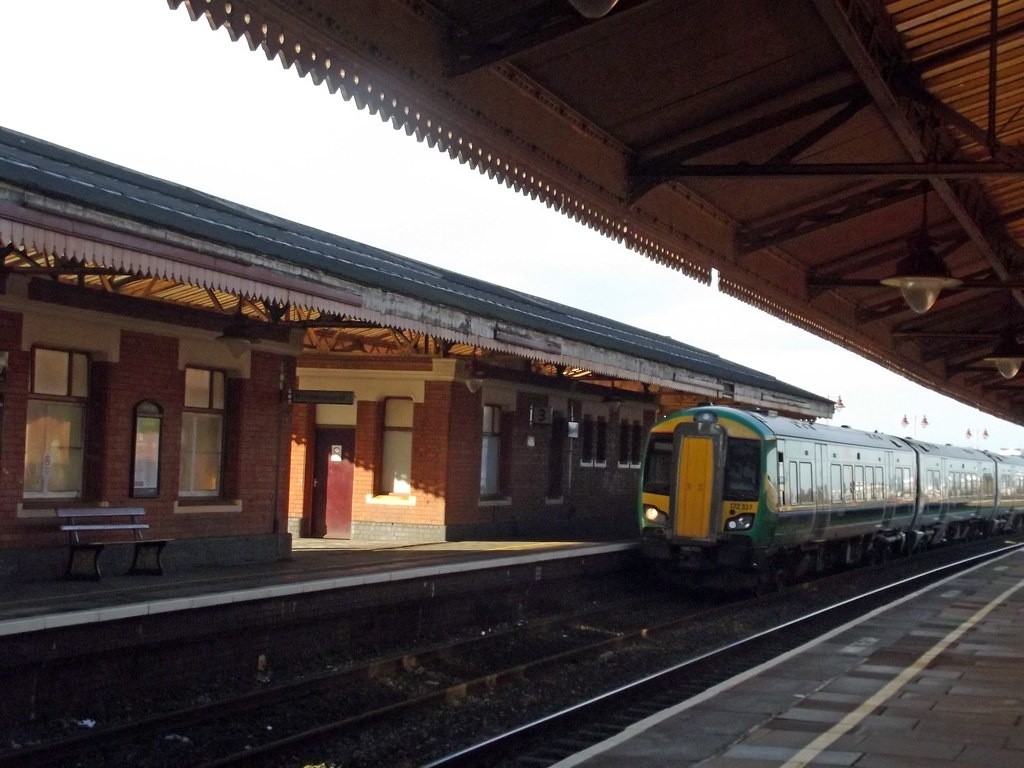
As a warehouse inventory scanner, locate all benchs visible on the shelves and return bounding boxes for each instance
[55,507,175,580]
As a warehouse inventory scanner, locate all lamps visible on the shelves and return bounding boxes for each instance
[881,179,962,313]
[463,347,487,394]
[216,292,262,358]
[984,289,1024,380]
[601,377,625,412]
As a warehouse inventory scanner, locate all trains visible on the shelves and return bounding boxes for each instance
[636,400,1024,605]
